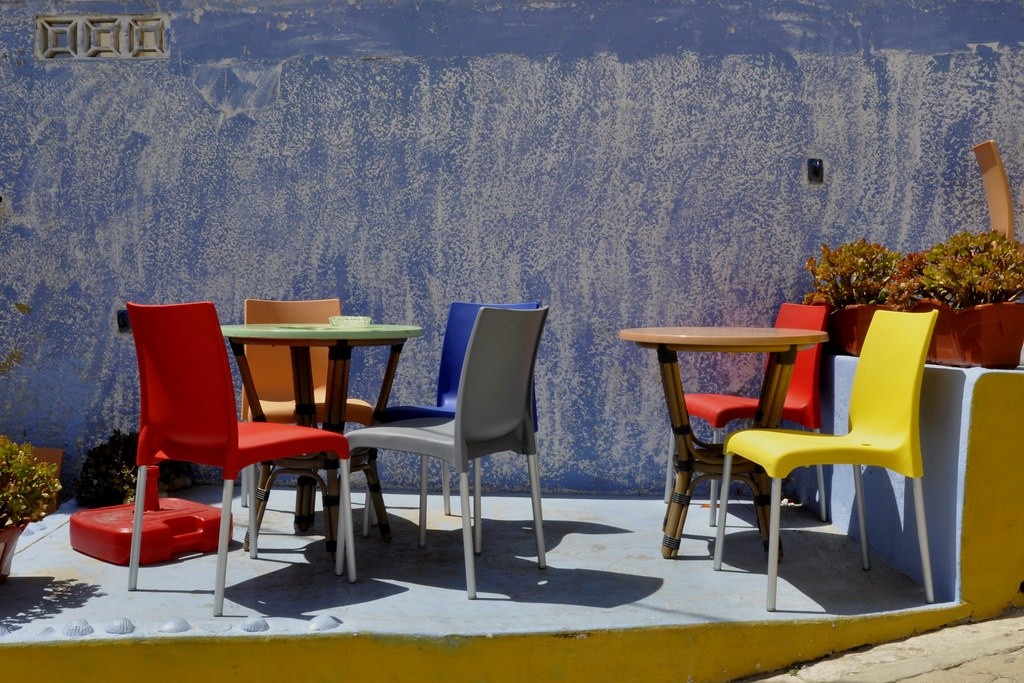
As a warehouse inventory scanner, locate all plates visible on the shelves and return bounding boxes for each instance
[327,316,371,326]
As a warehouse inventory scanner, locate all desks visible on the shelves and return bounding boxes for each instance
[218,321,426,554]
[617,325,827,563]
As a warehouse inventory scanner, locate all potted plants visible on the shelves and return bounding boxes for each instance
[870,229,1024,369]
[799,238,907,357]
[0,435,63,584]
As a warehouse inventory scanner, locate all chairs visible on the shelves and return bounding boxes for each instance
[334,306,552,602]
[122,301,357,618]
[662,302,828,528]
[241,299,375,540]
[712,308,940,613]
[360,301,543,555]
